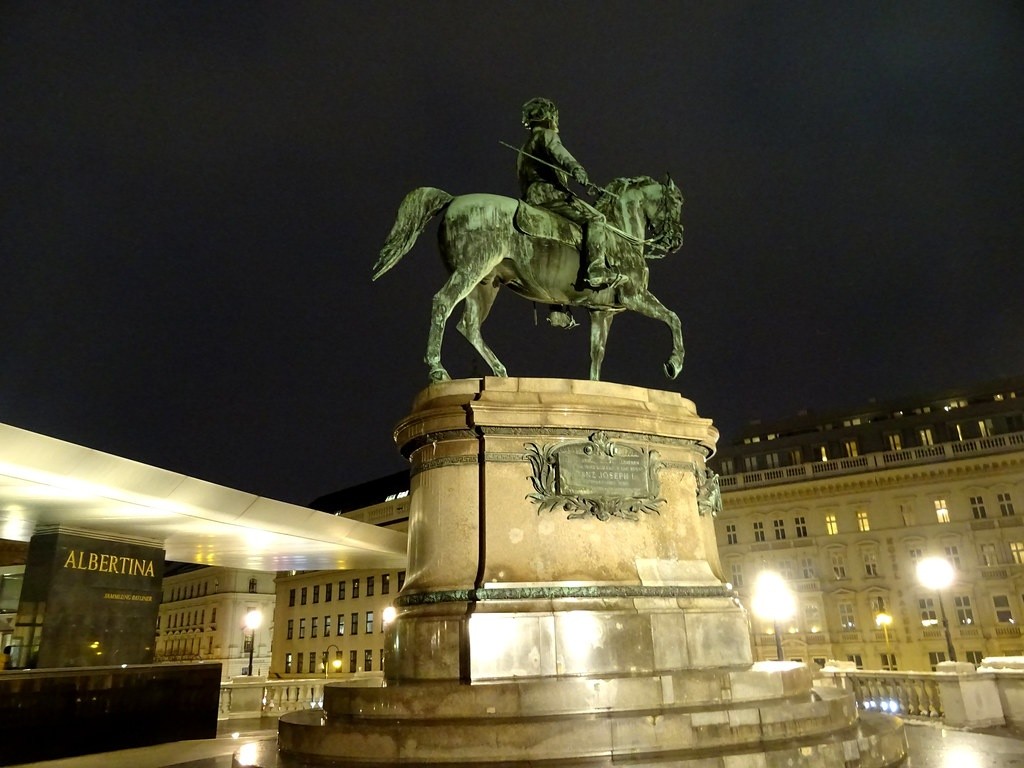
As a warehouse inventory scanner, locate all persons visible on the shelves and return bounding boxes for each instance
[518,99,629,329]
[0,646,12,670]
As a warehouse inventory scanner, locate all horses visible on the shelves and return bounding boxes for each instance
[372,171,686,382]
